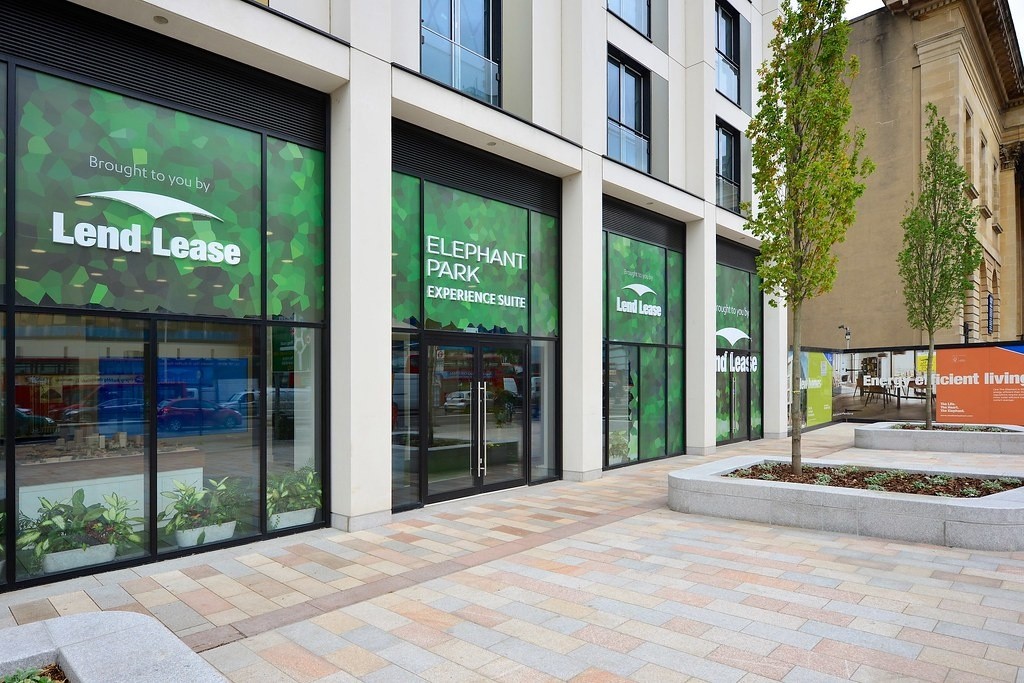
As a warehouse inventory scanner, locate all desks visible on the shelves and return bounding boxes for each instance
[15,451,204,525]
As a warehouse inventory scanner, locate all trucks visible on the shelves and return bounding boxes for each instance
[272,387,294,427]
[392,371,441,410]
[64,378,186,409]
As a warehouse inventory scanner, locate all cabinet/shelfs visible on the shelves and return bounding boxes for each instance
[862,357,879,376]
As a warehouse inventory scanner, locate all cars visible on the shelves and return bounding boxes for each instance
[509,391,523,406]
[0,400,57,438]
[63,398,144,423]
[486,391,506,410]
[157,396,243,432]
[444,390,482,414]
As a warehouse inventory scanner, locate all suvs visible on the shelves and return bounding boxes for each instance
[218,388,259,416]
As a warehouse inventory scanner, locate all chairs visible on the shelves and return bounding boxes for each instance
[852,373,891,410]
[906,380,935,406]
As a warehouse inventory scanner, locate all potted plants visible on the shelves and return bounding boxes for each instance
[267,467,321,529]
[17,488,149,573]
[157,477,237,547]
